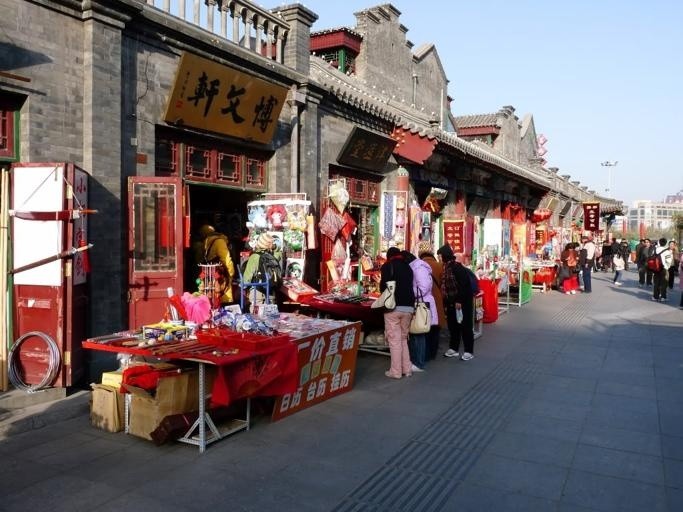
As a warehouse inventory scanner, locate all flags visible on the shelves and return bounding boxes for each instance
[583,202,600,232]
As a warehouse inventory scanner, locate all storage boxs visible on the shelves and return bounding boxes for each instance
[89,370,125,433]
[119,371,213,445]
[102,364,183,388]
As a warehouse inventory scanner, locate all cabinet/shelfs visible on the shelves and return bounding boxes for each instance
[82,320,300,455]
[209,297,362,423]
[236,196,311,302]
[463,251,562,341]
[283,286,391,359]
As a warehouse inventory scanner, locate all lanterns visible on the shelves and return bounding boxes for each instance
[531,208,552,222]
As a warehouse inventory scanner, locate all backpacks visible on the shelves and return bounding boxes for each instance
[464,266,478,294]
[252,250,282,291]
[647,247,668,273]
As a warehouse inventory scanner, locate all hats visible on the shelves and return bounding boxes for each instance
[257,234,274,250]
[580,236,588,240]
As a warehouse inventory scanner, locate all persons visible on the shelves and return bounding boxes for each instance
[417,242,444,362]
[623,242,631,271]
[611,238,619,258]
[562,244,579,294]
[638,239,652,288]
[619,238,626,250]
[678,254,683,310]
[602,239,611,272]
[667,241,677,291]
[651,238,672,301]
[612,247,625,286]
[380,247,416,379]
[240,231,284,314]
[581,236,596,294]
[636,239,644,266]
[399,250,438,371]
[574,242,580,253]
[192,223,235,303]
[436,244,474,361]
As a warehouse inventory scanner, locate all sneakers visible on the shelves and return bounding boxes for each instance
[564,291,570,296]
[443,349,459,357]
[410,363,423,373]
[651,296,657,301]
[385,370,401,379]
[582,290,591,294]
[460,352,473,362]
[661,298,670,302]
[571,289,577,295]
[401,371,412,377]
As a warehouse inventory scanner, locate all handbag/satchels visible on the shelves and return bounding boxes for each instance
[370,263,397,312]
[558,259,570,280]
[197,237,229,299]
[407,288,433,334]
